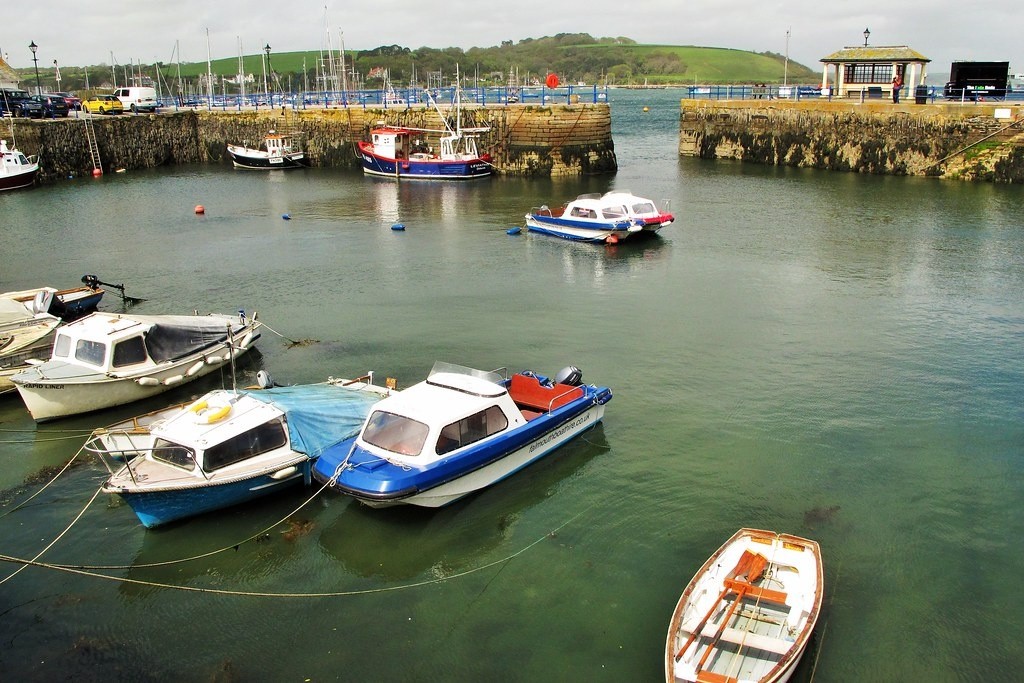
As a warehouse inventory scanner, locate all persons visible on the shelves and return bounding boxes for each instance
[892,74,901,104]
[753,83,766,99]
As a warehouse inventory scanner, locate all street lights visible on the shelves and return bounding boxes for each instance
[29,41,49,118]
[862,27,871,47]
[782,25,792,85]
[263,42,277,109]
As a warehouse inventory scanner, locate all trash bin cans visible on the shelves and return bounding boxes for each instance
[571,95,578,104]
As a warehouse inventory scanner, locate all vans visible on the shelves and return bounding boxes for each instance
[112,87,158,112]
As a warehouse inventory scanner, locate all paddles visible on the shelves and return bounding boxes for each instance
[695,554,768,674]
[674,549,756,663]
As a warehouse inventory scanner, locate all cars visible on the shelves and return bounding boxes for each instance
[797,85,820,96]
[81,93,123,114]
[31,94,69,117]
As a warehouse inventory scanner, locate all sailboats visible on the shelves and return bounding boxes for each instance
[53,15,608,103]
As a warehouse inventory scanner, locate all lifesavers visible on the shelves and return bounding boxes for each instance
[546,75,559,88]
[191,399,233,425]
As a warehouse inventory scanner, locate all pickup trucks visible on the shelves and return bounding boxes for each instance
[0,88,43,118]
[48,90,81,110]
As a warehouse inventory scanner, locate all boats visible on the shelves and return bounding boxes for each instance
[310,359,615,510]
[0,138,40,196]
[688,86,711,95]
[524,188,675,245]
[658,524,828,683]
[356,120,506,183]
[1006,66,1024,95]
[0,277,117,392]
[225,130,308,171]
[10,312,261,424]
[102,374,403,531]
[88,372,280,461]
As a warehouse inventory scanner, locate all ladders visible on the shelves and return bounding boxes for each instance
[86,118,103,174]
